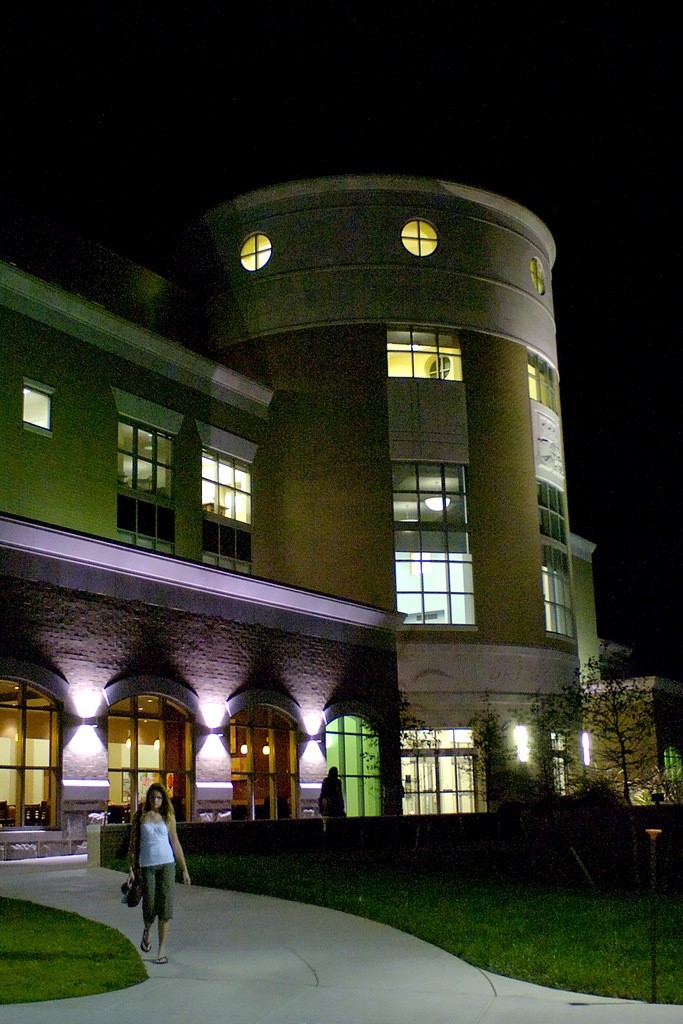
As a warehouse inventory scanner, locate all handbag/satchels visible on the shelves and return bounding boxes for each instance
[121,874,145,907]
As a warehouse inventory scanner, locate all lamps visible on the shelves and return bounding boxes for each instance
[398,505,418,533]
[309,733,321,743]
[424,482,451,511]
[209,727,224,736]
[82,717,98,728]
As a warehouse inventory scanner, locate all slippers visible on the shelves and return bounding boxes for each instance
[141,928,152,953]
[155,949,168,964]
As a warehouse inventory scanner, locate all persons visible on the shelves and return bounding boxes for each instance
[126,782,190,964]
[318,766,346,817]
